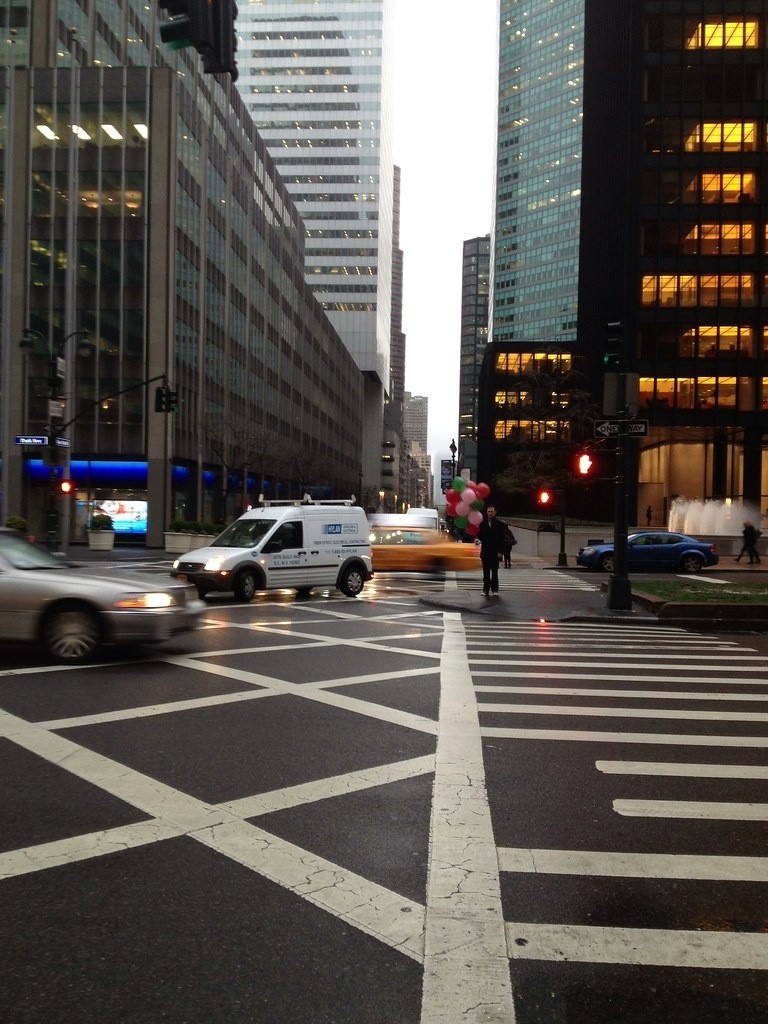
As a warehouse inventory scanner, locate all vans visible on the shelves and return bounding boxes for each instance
[170,498,375,605]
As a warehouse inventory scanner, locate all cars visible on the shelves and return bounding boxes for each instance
[575,532,719,572]
[367,514,484,573]
[0,525,206,662]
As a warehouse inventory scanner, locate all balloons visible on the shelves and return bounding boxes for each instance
[446,476,490,535]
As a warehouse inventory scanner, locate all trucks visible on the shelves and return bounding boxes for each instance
[406,507,448,532]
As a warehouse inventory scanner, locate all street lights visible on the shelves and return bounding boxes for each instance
[379,492,385,514]
[394,495,411,514]
[20,326,89,557]
[449,438,458,480]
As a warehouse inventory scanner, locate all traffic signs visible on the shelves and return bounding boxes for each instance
[595,420,650,438]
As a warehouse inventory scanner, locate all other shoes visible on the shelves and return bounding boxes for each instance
[754,560,761,564]
[492,590,502,595]
[746,562,753,564]
[481,591,488,596]
[733,558,739,562]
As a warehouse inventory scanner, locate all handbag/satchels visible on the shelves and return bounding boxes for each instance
[754,529,762,539]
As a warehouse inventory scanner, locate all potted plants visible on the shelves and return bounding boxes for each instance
[85,514,115,551]
[163,518,233,554]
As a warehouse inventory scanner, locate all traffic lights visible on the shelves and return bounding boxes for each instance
[164,386,176,413]
[537,490,555,507]
[58,480,74,494]
[576,450,608,479]
[154,386,166,414]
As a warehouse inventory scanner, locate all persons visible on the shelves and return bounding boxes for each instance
[475,506,506,596]
[91,501,134,520]
[503,524,518,569]
[734,521,762,564]
[646,505,653,526]
